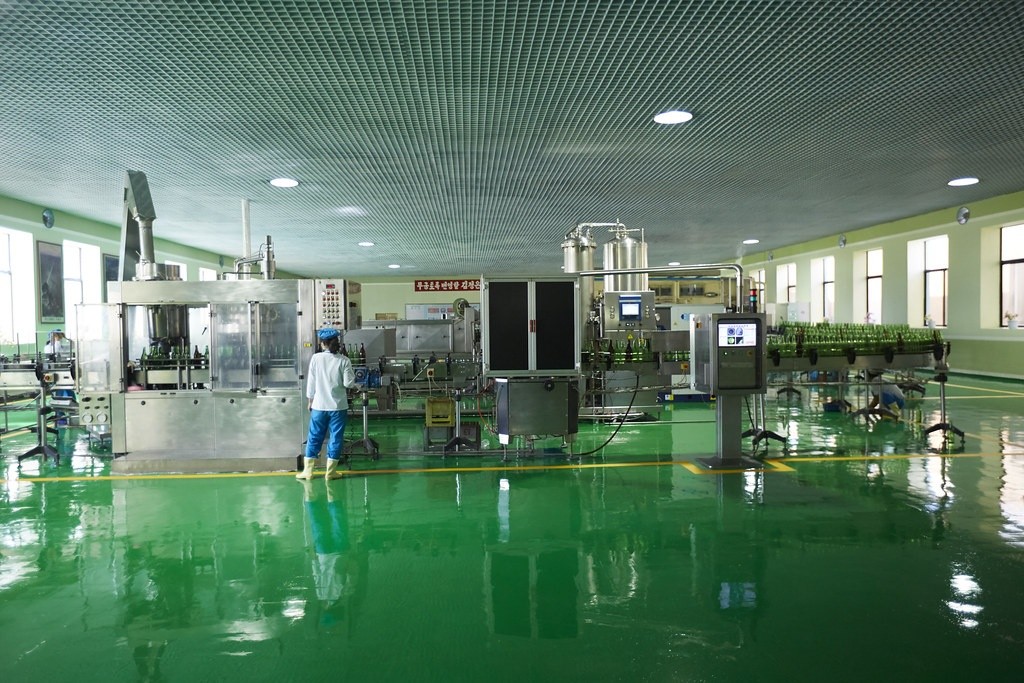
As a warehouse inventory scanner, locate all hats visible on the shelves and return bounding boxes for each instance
[318,327,340,340]
[49,329,61,338]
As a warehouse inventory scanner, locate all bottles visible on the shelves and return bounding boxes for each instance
[354,344,360,367]
[359,343,366,366]
[317,344,322,353]
[347,344,354,366]
[340,344,347,357]
[139,346,297,370]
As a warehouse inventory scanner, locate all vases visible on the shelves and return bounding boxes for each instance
[1008,321,1019,329]
[928,321,935,328]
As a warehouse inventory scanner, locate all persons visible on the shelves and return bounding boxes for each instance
[46,330,77,402]
[296,329,355,482]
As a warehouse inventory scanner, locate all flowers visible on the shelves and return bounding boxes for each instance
[1004,312,1019,320]
[864,312,875,323]
[923,314,933,321]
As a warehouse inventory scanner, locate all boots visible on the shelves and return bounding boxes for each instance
[296,457,316,480]
[296,479,316,502]
[325,481,339,502]
[325,457,342,480]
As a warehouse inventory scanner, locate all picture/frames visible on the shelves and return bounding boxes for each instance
[103,253,119,302]
[36,240,65,324]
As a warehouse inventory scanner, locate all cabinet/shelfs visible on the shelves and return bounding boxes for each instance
[480,273,582,377]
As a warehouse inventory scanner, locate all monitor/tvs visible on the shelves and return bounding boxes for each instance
[622,304,639,315]
[718,323,756,346]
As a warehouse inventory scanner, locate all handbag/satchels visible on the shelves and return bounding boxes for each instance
[69,361,82,379]
